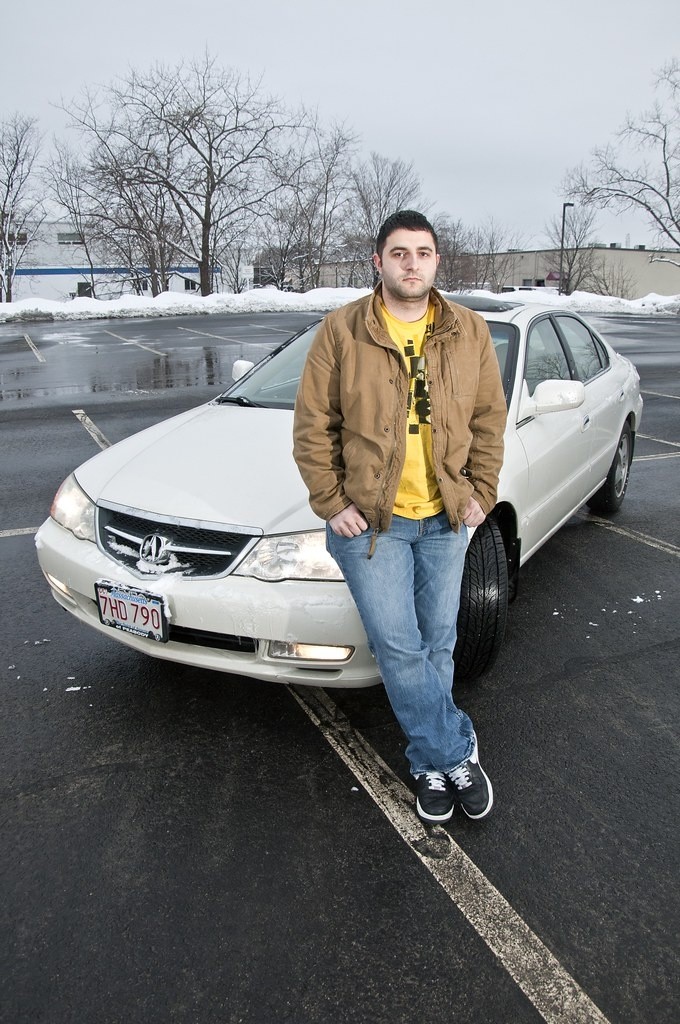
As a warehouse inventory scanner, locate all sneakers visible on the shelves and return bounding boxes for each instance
[444,730,493,819]
[416,772,454,824]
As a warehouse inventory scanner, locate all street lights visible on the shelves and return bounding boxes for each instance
[558,203,575,296]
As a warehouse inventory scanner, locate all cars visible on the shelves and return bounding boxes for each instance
[34,294,641,689]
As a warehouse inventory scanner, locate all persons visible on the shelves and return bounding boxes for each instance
[292,209,508,820]
